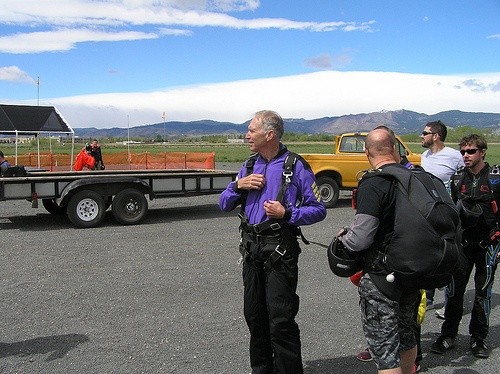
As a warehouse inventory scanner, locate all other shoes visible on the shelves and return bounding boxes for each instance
[357,347,373,361]
[435,306,445,318]
[425,298,434,311]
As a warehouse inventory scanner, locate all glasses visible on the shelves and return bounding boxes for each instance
[459,147,482,155]
[422,130,441,136]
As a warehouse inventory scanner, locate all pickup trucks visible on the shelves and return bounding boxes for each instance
[297,131,422,209]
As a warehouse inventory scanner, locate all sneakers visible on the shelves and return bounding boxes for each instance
[470,334,488,356]
[429,335,456,354]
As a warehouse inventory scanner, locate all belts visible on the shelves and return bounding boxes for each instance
[241,230,279,243]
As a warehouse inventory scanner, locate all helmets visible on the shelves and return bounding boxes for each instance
[327,228,364,278]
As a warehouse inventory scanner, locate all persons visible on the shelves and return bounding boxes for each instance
[0,150,10,177]
[217,109,328,374]
[336,118,500,374]
[85,138,104,171]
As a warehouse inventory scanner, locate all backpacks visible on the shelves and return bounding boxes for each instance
[355,164,462,292]
